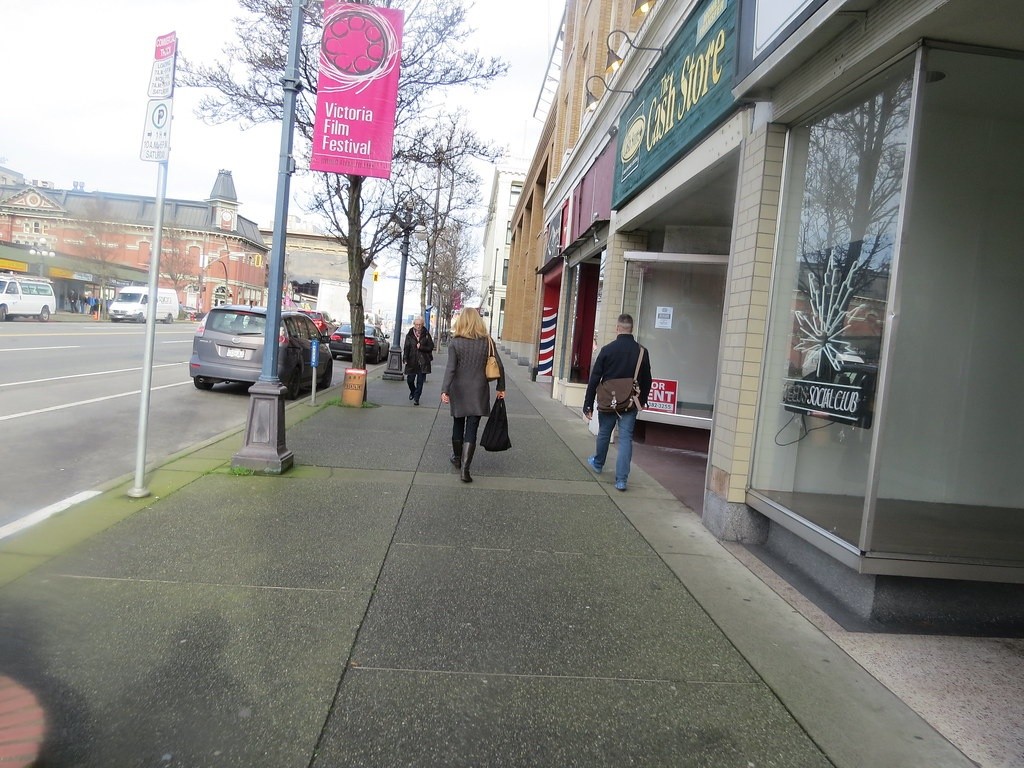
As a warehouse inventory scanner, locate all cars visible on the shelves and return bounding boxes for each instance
[329,324,389,363]
[787,362,878,430]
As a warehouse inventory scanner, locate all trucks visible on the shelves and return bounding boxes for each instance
[109,286,180,323]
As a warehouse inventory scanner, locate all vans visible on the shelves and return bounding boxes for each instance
[0,271,56,322]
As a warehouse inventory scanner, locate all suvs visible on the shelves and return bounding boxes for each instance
[188,304,333,400]
[299,310,338,337]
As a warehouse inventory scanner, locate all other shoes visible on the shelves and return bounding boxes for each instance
[409,392,414,400]
[414,401,419,405]
[614,480,627,490]
[588,453,602,474]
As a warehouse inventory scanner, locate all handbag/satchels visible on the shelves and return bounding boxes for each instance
[480,396,512,452]
[486,335,500,381]
[596,346,644,419]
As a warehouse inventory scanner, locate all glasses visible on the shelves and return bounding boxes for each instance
[415,324,422,327]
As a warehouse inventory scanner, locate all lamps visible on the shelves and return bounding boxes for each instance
[632,0,656,19]
[606,30,664,76]
[584,75,634,112]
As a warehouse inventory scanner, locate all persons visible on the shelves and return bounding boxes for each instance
[68,289,95,314]
[582,313,652,491]
[440,308,505,483]
[403,315,434,405]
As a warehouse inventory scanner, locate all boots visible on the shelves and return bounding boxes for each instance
[450,436,463,469]
[461,442,473,482]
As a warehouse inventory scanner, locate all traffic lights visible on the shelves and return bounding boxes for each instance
[373,272,378,281]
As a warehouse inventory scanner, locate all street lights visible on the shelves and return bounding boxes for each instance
[382,200,407,381]
[29,237,55,276]
